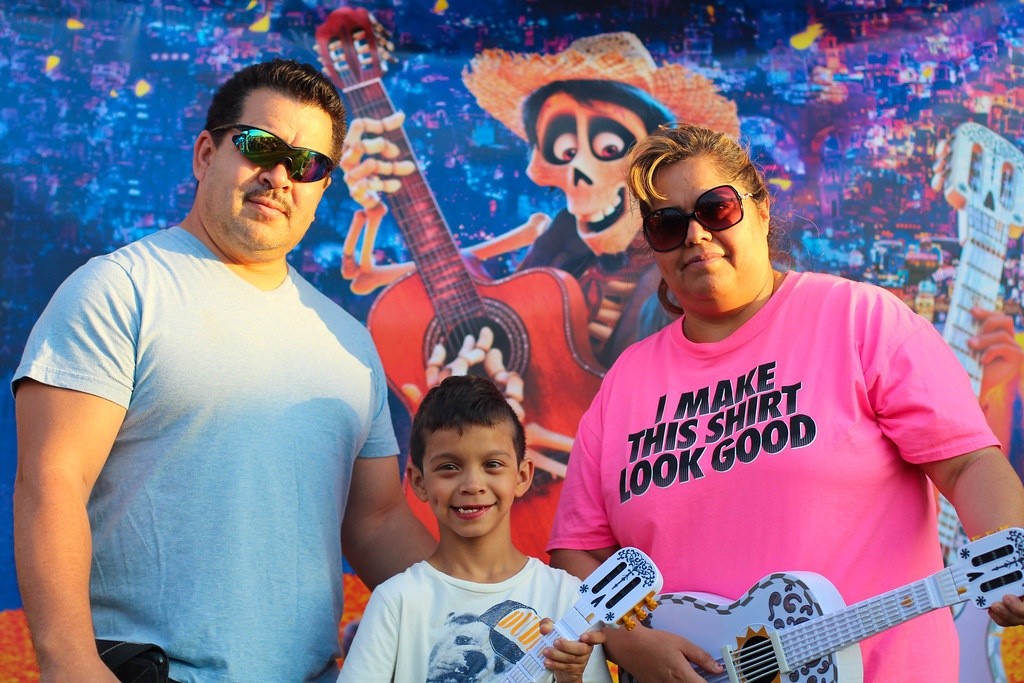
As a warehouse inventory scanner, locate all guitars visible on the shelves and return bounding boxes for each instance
[494,546,664,683]
[616,523,1024,683]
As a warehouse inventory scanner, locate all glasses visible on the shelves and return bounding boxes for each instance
[210,123,333,182]
[642,185,763,252]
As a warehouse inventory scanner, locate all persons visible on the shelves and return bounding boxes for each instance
[10,58,437,683]
[335,375,611,683]
[547,123,1024,683]
